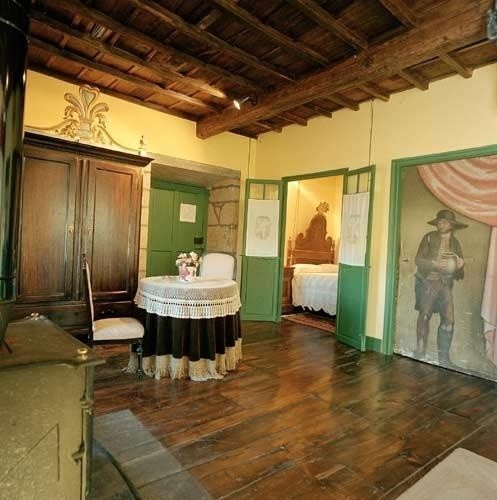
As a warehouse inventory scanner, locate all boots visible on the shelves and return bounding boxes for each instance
[437,325,465,372]
[413,320,431,360]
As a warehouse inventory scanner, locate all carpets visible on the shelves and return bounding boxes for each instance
[282,312,336,334]
[395,448,497,499]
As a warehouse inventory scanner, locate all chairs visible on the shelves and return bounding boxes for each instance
[199,252,235,280]
[81,262,146,381]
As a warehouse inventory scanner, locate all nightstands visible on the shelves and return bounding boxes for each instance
[281,265,294,314]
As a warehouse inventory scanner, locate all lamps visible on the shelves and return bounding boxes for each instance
[233,97,251,111]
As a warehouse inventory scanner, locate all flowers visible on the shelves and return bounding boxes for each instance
[175,250,200,267]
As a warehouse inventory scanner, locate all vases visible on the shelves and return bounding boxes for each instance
[178,267,197,282]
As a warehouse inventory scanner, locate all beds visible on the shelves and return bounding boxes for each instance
[286,214,340,319]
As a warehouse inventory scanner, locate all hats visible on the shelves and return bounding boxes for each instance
[426,208,471,231]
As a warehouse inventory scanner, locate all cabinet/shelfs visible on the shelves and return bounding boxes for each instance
[0,313,105,500]
[11,130,155,334]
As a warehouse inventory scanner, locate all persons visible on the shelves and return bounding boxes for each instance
[412,210,469,371]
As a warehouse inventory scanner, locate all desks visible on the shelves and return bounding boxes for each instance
[134,273,243,381]
